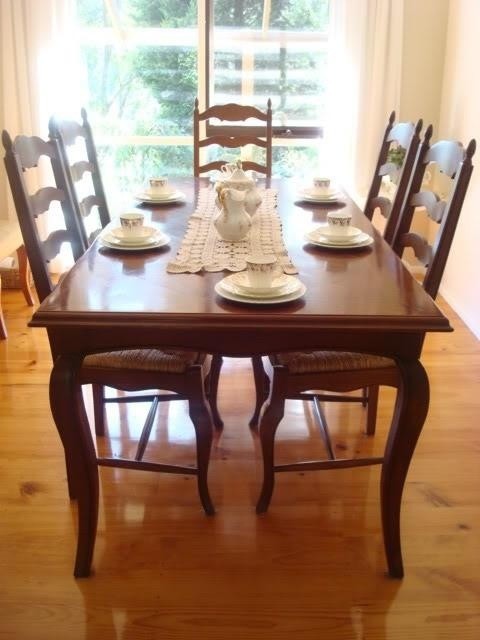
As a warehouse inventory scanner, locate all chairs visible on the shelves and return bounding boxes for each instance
[244,119,478,580]
[187,92,277,177]
[2,113,226,521]
[42,107,226,441]
[243,107,427,440]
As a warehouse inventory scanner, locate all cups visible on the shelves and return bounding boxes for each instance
[120,214,145,236]
[246,253,288,286]
[149,177,169,193]
[327,213,353,234]
[313,178,330,193]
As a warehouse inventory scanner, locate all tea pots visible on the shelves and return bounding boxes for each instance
[214,188,254,242]
[214,160,262,223]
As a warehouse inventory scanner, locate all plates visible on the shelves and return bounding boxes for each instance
[98,226,172,252]
[305,232,375,249]
[297,187,347,203]
[133,186,186,205]
[212,271,306,304]
[315,224,363,242]
